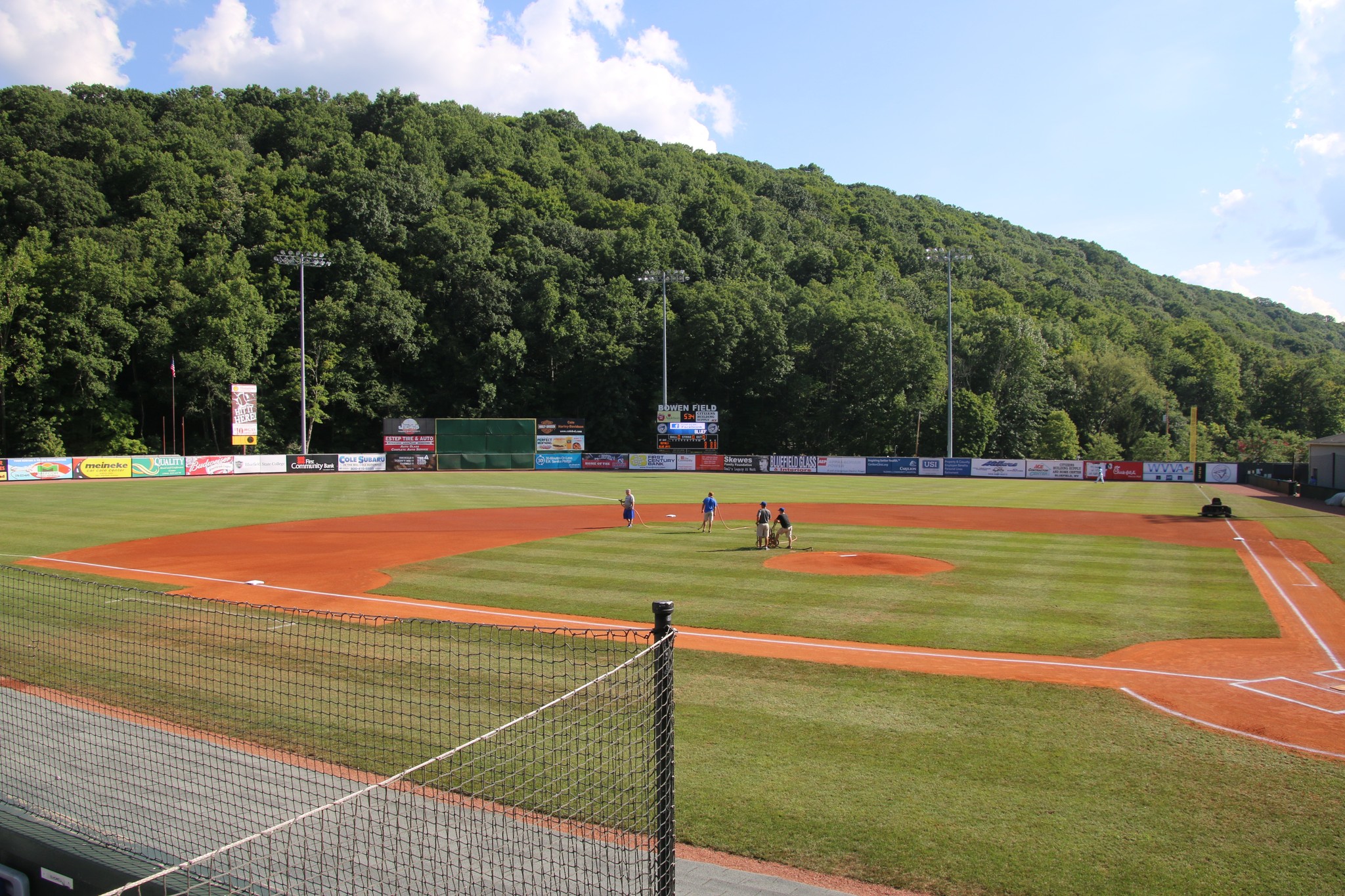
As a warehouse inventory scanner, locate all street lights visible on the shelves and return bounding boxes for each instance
[273,250,332,454]
[925,247,973,459]
[638,268,690,407]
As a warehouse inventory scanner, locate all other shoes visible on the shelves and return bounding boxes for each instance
[757,548,762,549]
[703,530,705,533]
[786,545,792,549]
[627,524,632,527]
[709,531,711,533]
[765,547,770,550]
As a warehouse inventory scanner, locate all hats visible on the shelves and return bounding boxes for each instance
[760,502,767,505]
[777,508,785,512]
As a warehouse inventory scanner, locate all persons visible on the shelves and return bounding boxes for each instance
[755,456,769,472]
[621,489,635,527]
[702,492,717,533]
[773,507,793,549]
[755,501,771,550]
[1309,477,1317,486]
